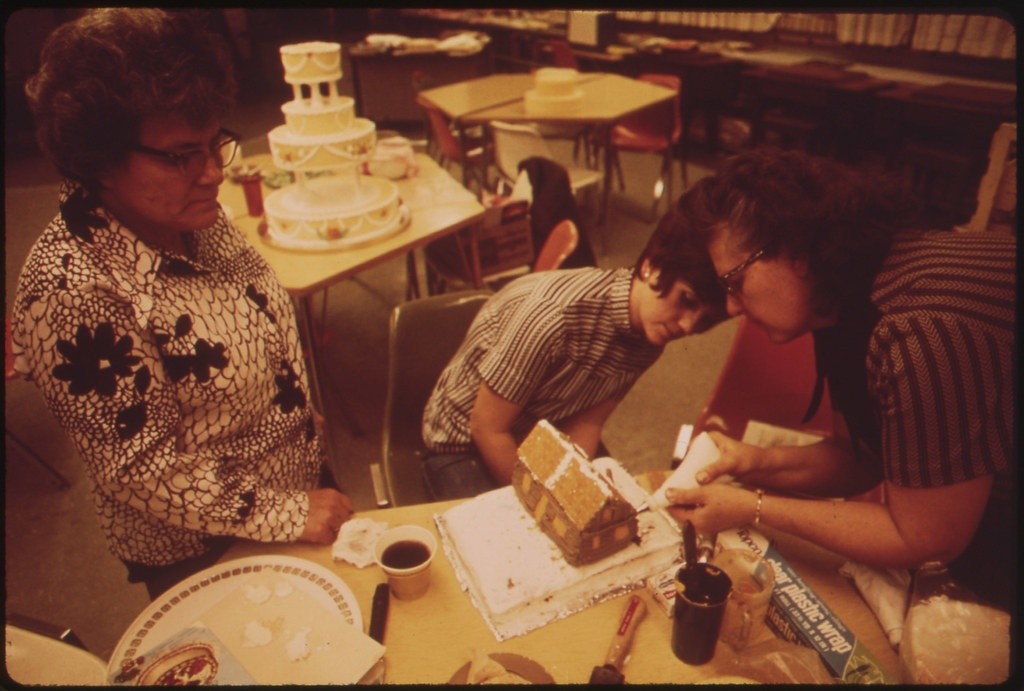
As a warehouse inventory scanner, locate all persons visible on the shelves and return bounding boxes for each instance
[663,147,1019,613]
[416,192,736,502]
[11,8,354,604]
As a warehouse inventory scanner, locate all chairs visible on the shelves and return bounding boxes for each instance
[598,69,687,225]
[486,119,607,234]
[410,93,495,189]
[7,310,69,494]
[522,39,587,168]
[369,290,496,508]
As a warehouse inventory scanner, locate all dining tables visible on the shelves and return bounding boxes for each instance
[204,481,911,684]
[218,125,483,448]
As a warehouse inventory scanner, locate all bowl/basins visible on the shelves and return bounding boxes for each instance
[366,136,413,178]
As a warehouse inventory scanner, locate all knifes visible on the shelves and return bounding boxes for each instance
[359,584,390,685]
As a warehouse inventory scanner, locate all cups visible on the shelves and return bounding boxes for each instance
[230,166,263,218]
[671,562,733,668]
[714,547,774,652]
[374,524,436,601]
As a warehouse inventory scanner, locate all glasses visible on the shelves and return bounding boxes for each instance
[125,129,241,180]
[720,238,777,294]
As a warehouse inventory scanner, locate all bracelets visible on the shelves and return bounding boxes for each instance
[753,489,763,530]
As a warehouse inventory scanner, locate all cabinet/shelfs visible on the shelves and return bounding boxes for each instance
[350,29,495,127]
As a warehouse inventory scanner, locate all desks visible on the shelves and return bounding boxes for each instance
[420,71,600,190]
[468,73,680,250]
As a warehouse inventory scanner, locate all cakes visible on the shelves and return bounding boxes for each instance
[524,65,585,117]
[440,420,683,623]
[261,40,403,248]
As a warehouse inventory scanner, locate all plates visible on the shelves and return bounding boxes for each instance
[104,553,364,688]
